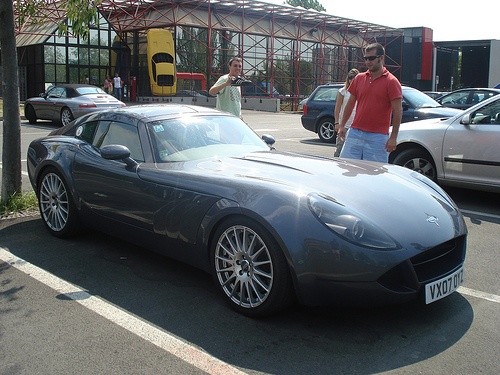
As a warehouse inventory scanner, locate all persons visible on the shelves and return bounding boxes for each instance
[334,68,360,157]
[338,42,403,163]
[104,71,123,101]
[209,57,242,119]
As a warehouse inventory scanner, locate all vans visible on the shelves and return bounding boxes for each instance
[240,82,279,100]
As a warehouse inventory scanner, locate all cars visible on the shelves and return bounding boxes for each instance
[24,84,125,128]
[434,88,500,112]
[389,92,500,202]
[301,83,465,144]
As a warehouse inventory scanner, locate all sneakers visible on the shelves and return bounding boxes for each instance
[230,78,252,85]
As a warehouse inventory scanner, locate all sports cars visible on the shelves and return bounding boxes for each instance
[27,102,468,321]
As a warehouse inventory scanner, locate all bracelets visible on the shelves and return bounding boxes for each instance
[334,122,339,124]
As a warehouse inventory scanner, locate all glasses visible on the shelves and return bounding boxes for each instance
[363,56,379,61]
[348,75,353,80]
